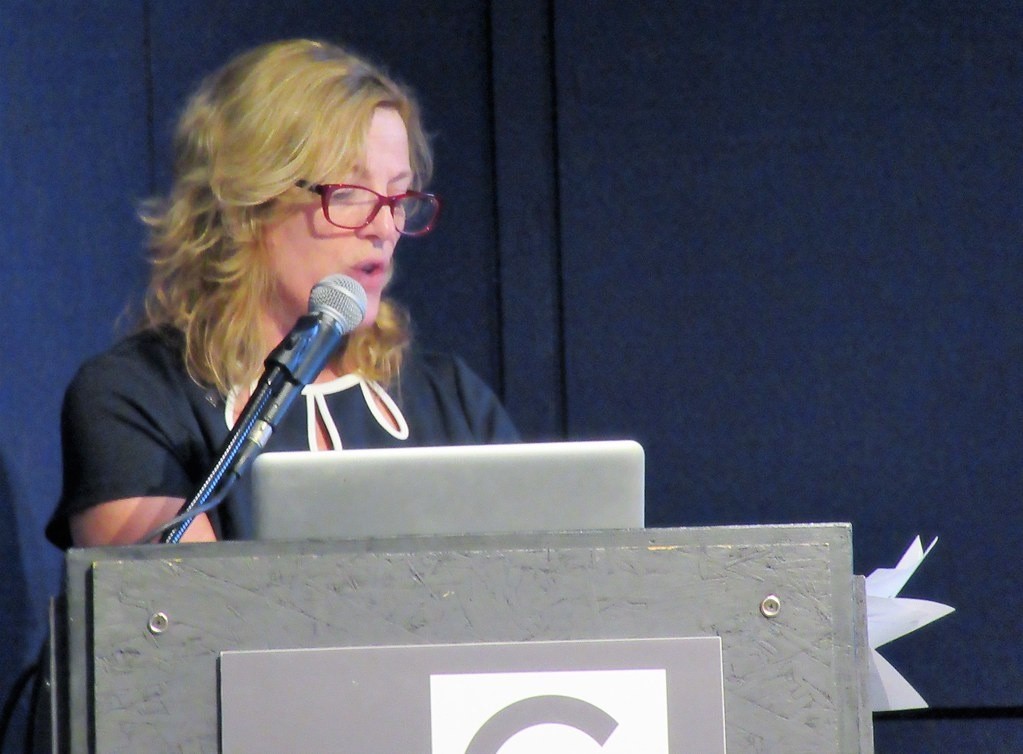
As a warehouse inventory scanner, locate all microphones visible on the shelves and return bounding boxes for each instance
[230,273,368,478]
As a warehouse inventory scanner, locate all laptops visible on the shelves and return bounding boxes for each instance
[252,439,645,544]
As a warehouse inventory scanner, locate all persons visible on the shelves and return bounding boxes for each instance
[41,37,526,549]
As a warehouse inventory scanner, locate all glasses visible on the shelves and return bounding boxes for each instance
[294,177,441,236]
